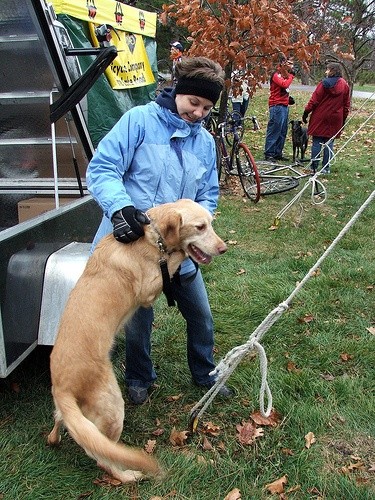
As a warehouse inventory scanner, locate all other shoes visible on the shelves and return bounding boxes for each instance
[274,156,289,162]
[126,380,151,405]
[194,380,233,399]
[237,122,243,128]
[321,169,330,173]
[234,123,238,129]
[264,155,276,163]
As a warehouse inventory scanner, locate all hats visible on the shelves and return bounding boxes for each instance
[169,42,184,52]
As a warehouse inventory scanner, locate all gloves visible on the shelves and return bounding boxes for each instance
[303,111,309,124]
[111,206,151,244]
[289,69,297,76]
[288,96,295,105]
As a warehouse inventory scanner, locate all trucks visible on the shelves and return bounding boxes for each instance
[0,0,162,378]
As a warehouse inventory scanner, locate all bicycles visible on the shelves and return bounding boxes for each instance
[229,159,325,196]
[201,96,261,204]
[156,59,177,95]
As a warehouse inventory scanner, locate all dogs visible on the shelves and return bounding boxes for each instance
[288,119,309,163]
[43,197,230,485]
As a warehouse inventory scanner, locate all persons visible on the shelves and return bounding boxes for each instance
[228,57,251,134]
[168,42,184,85]
[302,62,350,174]
[85,54,238,404]
[262,60,294,163]
